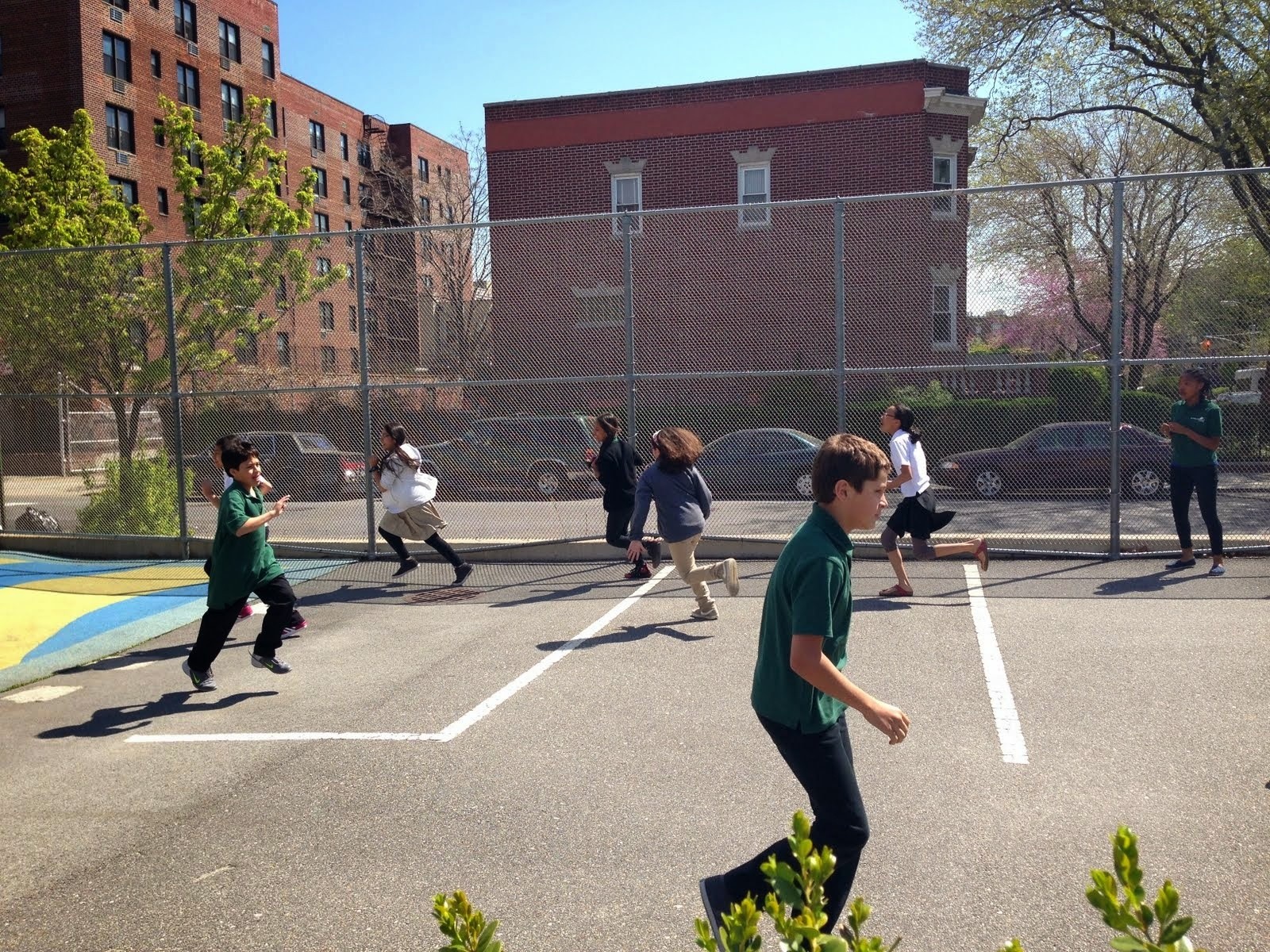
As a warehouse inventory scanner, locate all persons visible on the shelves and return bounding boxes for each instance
[699,433,908,950]
[587,414,660,581]
[182,434,308,692]
[1158,367,1226,575]
[625,427,739,622]
[879,403,989,597]
[368,421,474,589]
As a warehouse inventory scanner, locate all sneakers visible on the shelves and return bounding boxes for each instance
[452,563,474,586]
[722,559,739,597]
[692,608,718,619]
[234,605,253,624]
[647,540,661,568]
[182,660,217,691]
[280,619,307,639]
[624,562,651,577]
[251,653,292,674]
[392,557,420,578]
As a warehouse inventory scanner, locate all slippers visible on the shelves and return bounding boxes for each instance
[879,584,913,596]
[973,539,988,571]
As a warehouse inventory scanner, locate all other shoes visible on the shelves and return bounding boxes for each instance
[1165,557,1196,569]
[1209,564,1225,575]
[700,874,741,952]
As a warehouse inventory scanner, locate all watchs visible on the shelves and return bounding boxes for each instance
[368,466,378,473]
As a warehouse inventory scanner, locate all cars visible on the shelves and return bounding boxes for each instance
[187,431,362,499]
[420,412,597,500]
[940,420,1169,505]
[692,427,823,497]
[1231,368,1270,407]
